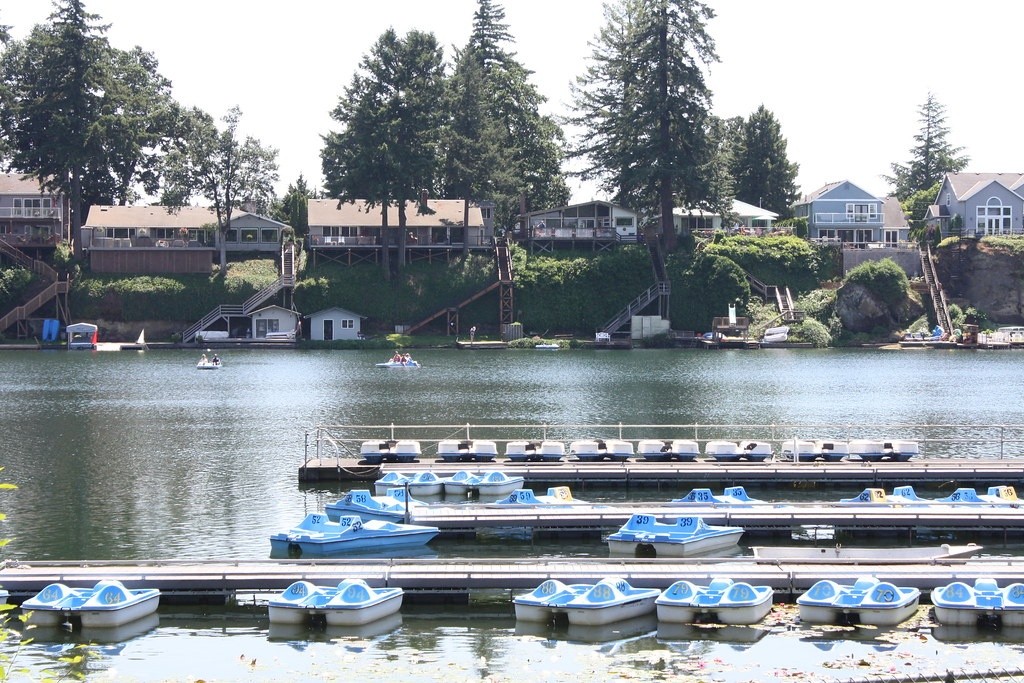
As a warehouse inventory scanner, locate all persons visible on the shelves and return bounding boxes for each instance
[200,354,208,364]
[538,220,546,228]
[212,353,221,365]
[470,326,476,342]
[741,223,784,236]
[85,332,90,339]
[733,220,740,234]
[702,229,714,234]
[844,239,850,247]
[390,351,411,368]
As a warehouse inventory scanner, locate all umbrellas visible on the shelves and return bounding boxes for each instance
[753,216,777,227]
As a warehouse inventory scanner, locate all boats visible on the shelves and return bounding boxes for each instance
[323,486,427,525]
[795,576,921,626]
[929,578,1024,629]
[655,618,770,653]
[570,439,635,461]
[654,577,775,626]
[635,439,701,463]
[670,486,793,509]
[747,545,984,566]
[436,441,498,462]
[268,577,405,627]
[0,589,11,605]
[782,438,850,463]
[268,512,441,554]
[267,611,403,653]
[535,343,560,350]
[604,514,745,555]
[196,361,223,369]
[838,486,1024,512]
[492,485,606,510]
[850,438,918,463]
[441,471,526,495]
[372,470,449,498]
[66,322,97,349]
[931,625,1024,645]
[17,580,162,630]
[513,612,658,653]
[504,442,567,463]
[704,441,772,462]
[375,359,418,368]
[977,326,1024,349]
[360,440,422,464]
[510,577,663,627]
[23,612,160,657]
